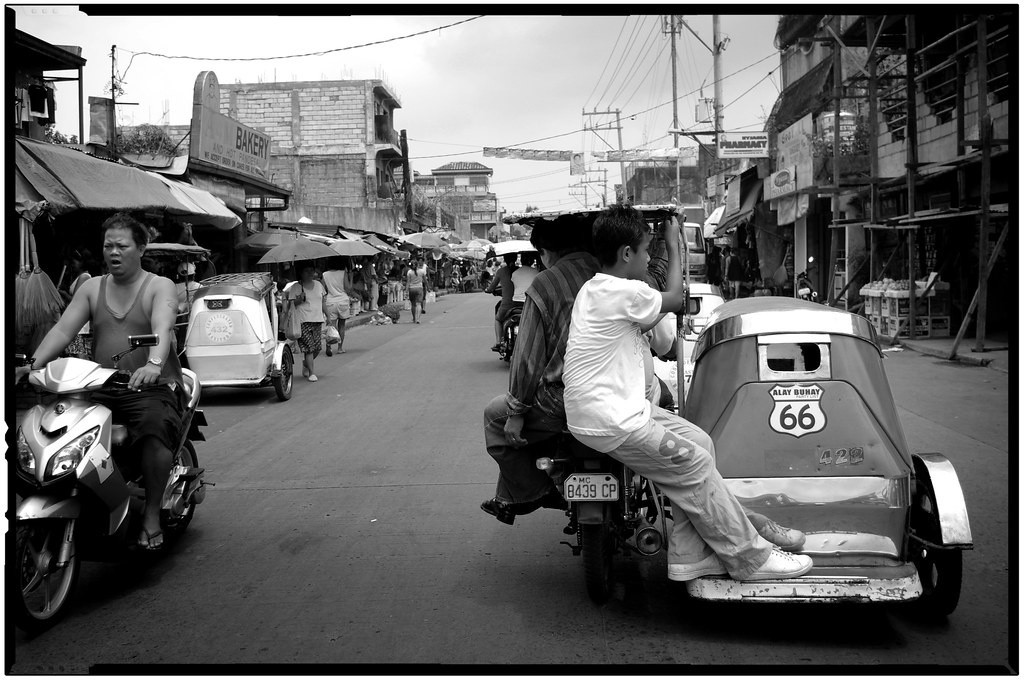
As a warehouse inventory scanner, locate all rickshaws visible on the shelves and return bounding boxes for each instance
[535,282,974,607]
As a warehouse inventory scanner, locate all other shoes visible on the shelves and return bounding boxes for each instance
[326,348,333,357]
[302,362,310,377]
[515,488,557,515]
[371,307,378,311]
[337,349,345,354]
[492,342,504,351]
[308,374,317,382]
[421,310,425,314]
[480,499,516,526]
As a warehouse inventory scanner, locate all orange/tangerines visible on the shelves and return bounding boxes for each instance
[863,278,894,290]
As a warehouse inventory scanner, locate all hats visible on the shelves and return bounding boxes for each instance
[178,263,194,276]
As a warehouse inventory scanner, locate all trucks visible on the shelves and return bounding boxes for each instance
[680,222,708,282]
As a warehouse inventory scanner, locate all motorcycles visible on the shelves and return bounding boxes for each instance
[786,256,820,302]
[485,286,522,361]
[14,334,215,636]
[183,273,295,400]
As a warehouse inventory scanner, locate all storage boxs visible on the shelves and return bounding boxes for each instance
[859,283,952,340]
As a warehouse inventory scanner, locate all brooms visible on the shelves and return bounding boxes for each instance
[15,218,66,340]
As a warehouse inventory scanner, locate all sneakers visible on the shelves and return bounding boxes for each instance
[742,545,812,580]
[758,518,806,549]
[668,548,728,581]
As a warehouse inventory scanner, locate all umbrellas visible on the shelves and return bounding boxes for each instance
[256,233,339,302]
[330,239,381,256]
[400,231,493,272]
[234,228,299,249]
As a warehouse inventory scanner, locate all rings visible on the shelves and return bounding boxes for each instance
[511,438,515,441]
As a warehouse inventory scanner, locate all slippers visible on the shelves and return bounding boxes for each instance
[139,527,163,550]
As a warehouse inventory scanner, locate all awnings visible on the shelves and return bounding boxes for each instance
[149,173,242,228]
[15,139,190,215]
[704,206,737,239]
[713,179,764,236]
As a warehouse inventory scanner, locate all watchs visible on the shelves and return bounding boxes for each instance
[148,358,163,368]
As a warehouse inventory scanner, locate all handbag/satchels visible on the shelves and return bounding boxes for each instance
[284,300,302,339]
[325,325,341,344]
[404,298,411,310]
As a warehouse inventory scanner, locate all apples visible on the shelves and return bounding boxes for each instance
[889,279,909,290]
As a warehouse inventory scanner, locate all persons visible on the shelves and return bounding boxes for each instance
[363,255,380,311]
[486,245,539,353]
[174,261,204,322]
[561,204,812,578]
[645,316,677,410]
[706,245,745,298]
[397,257,430,323]
[282,261,332,382]
[321,256,354,356]
[481,213,668,525]
[59,247,94,335]
[16,212,185,552]
[451,261,480,291]
